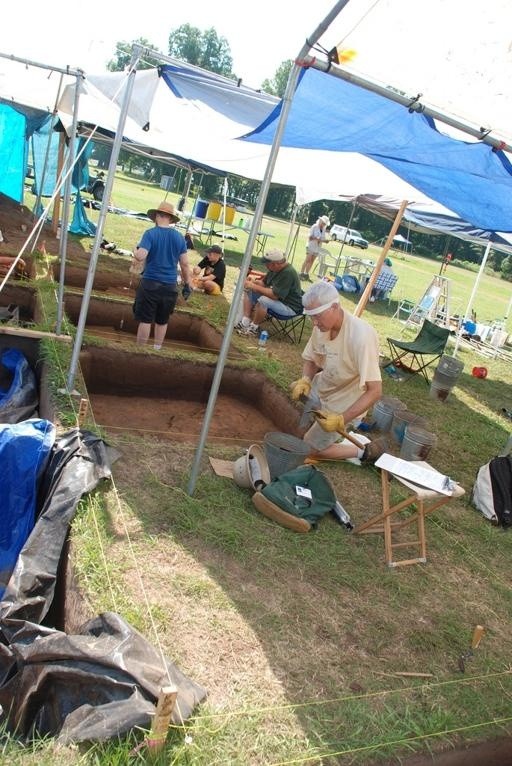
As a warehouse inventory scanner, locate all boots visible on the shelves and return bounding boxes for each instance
[368,436,394,482]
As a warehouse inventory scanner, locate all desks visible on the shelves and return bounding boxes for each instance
[174,209,274,257]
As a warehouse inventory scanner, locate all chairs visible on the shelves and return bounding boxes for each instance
[382,318,450,387]
[258,310,307,345]
[373,271,399,307]
[312,247,336,277]
[392,287,415,323]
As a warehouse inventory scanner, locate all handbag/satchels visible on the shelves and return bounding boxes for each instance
[252,465,337,533]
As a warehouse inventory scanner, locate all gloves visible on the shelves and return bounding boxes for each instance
[290,376,312,402]
[182,284,191,302]
[315,411,346,433]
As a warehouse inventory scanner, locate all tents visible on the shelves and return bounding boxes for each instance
[56,0,511,496]
[370,233,412,255]
[63,123,227,259]
[0,52,84,335]
[336,193,512,357]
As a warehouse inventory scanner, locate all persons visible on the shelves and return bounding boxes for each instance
[134,201,192,350]
[291,281,396,481]
[234,248,303,338]
[299,215,330,280]
[192,245,225,293]
[366,257,392,304]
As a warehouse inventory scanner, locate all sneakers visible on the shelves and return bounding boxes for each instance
[298,273,306,281]
[307,274,313,283]
[235,321,246,329]
[238,327,259,337]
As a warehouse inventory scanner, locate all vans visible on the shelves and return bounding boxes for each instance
[329,223,369,250]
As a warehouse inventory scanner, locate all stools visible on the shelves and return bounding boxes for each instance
[351,459,466,566]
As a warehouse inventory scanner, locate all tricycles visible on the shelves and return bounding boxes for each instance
[22,162,106,200]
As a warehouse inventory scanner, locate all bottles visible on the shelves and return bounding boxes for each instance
[238,218,243,228]
[257,329,269,347]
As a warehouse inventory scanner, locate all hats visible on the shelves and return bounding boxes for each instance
[260,248,286,265]
[385,258,393,267]
[232,443,270,491]
[204,244,223,253]
[147,202,181,224]
[319,215,331,225]
